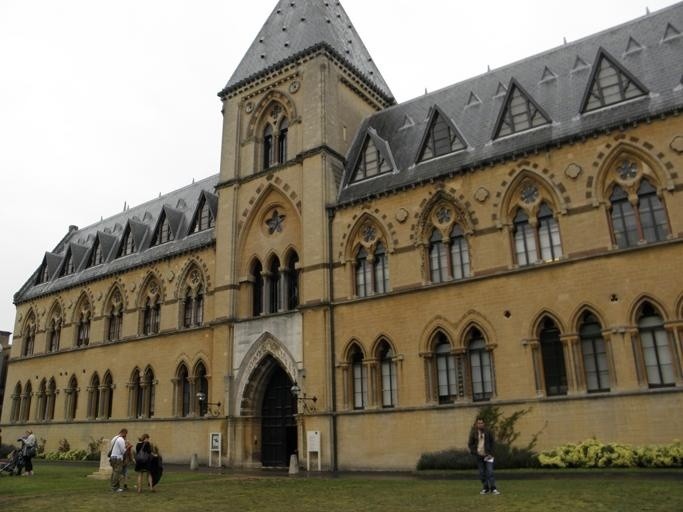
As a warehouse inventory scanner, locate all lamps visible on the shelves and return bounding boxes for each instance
[291,379,317,404]
[196,389,221,409]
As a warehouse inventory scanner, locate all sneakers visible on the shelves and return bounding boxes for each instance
[479,488,500,495]
[20,471,34,476]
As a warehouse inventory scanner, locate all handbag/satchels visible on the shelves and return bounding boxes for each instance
[134,442,150,463]
[26,445,36,458]
[106,449,112,457]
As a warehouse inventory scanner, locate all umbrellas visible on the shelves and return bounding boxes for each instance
[148,456,163,487]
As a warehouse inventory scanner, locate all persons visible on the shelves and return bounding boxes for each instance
[21,428,37,476]
[467,416,500,496]
[108,428,158,493]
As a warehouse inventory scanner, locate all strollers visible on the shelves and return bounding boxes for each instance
[0,447,24,475]
